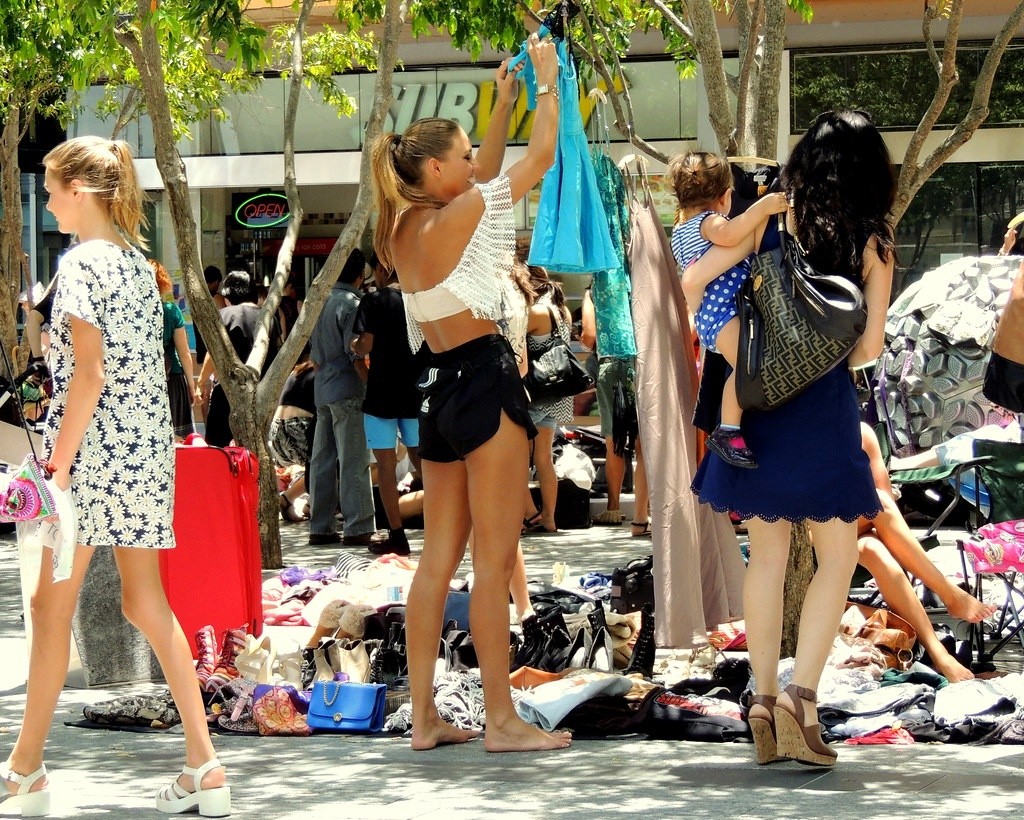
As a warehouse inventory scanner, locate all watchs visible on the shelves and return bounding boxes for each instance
[535,84,559,104]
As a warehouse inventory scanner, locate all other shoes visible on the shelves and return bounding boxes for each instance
[309,533,341,545]
[368,528,411,555]
[191,599,656,694]
[342,533,373,546]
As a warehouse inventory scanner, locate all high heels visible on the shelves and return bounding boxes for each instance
[154,758,232,818]
[1,764,53,816]
[773,685,839,768]
[747,695,790,766]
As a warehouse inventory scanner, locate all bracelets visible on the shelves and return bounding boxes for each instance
[999,248,1009,256]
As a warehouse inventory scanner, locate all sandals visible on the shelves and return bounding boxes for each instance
[279,494,312,522]
[522,511,542,532]
[630,521,652,536]
[523,526,557,536]
[593,510,623,525]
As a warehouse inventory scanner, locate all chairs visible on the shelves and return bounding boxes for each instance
[849,422,998,622]
[956,439,1023,672]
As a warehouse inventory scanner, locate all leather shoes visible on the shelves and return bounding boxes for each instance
[706,424,759,470]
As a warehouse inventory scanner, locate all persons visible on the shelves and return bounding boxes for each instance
[671,151,788,469]
[150,258,317,521]
[517,263,574,534]
[996,227,1018,256]
[857,421,992,683]
[369,30,572,751]
[580,290,652,537]
[308,248,377,545]
[0,134,231,820]
[18,281,58,375]
[351,251,421,555]
[681,107,895,766]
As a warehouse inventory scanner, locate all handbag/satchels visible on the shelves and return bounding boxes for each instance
[306,679,387,733]
[0,456,58,525]
[841,604,917,672]
[736,214,865,412]
[523,302,595,404]
